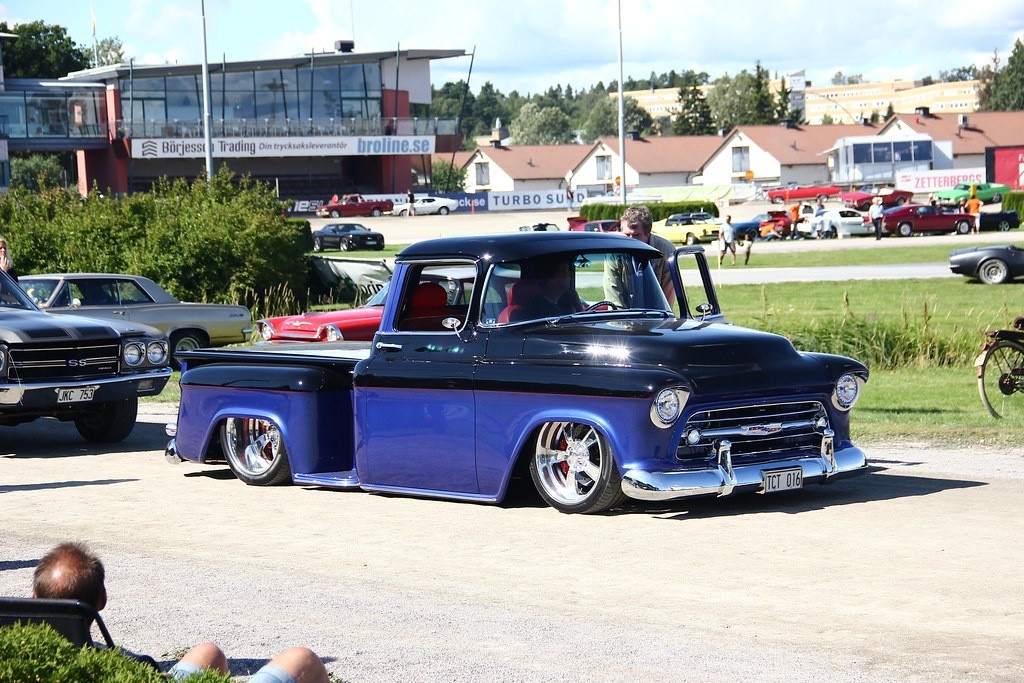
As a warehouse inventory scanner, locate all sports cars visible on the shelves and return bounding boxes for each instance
[516,178,1024,244]
[949,244,1024,285]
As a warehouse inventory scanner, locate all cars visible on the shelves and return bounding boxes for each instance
[0,268,174,445]
[312,223,385,253]
[253,264,610,343]
[383,197,458,217]
[15,272,254,371]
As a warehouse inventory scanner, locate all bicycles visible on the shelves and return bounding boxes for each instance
[974,316,1024,422]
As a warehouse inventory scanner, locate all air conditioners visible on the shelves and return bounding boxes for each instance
[959,115,970,125]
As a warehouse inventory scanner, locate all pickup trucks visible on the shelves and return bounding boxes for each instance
[165,231,872,515]
[316,193,394,218]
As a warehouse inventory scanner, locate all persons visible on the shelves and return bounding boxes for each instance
[845,199,861,210]
[738,234,753,266]
[602,204,677,327]
[812,197,825,216]
[928,194,937,206]
[406,189,415,217]
[0,235,19,304]
[28,288,44,304]
[331,195,355,204]
[32,539,332,683]
[959,195,984,235]
[517,259,579,322]
[50,289,69,307]
[91,289,109,305]
[869,197,885,241]
[787,199,802,240]
[718,215,741,268]
[760,221,782,242]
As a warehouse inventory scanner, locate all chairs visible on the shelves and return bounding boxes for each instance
[405,284,466,333]
[165,117,381,136]
[497,278,540,324]
[80,282,115,304]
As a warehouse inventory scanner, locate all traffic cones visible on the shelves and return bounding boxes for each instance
[469,199,477,214]
